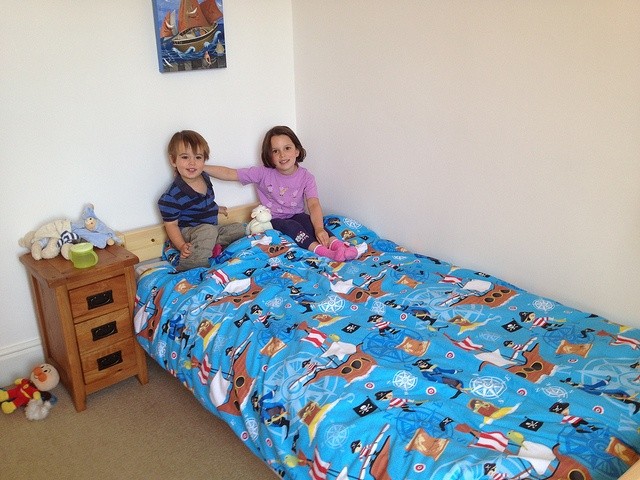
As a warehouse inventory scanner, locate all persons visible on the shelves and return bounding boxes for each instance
[159,130,245,273]
[173,126,358,262]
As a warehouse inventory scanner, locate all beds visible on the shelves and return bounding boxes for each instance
[123,204,640,476]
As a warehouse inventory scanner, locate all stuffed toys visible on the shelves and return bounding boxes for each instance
[245,205,273,236]
[1,363,60,419]
[18,204,124,260]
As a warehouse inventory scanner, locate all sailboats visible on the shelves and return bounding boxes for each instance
[160,0,224,53]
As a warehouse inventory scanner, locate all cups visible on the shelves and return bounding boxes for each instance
[68,243,98,269]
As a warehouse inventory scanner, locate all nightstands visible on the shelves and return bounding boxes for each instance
[19,245,148,412]
